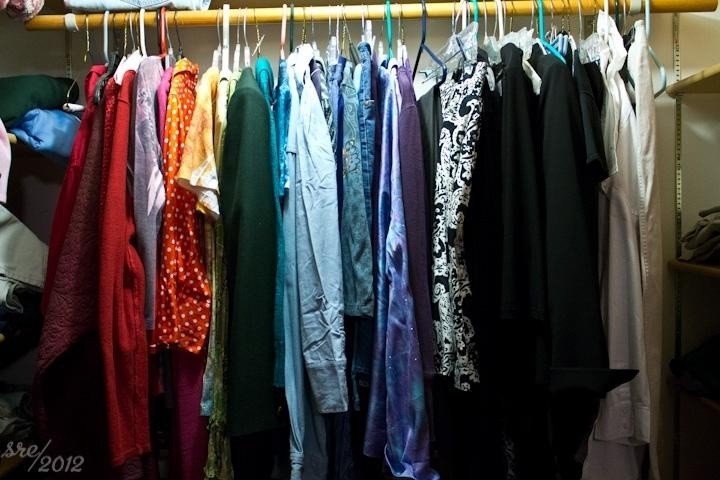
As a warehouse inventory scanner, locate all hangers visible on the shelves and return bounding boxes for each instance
[63,0,669,114]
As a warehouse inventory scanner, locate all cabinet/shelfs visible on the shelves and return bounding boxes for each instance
[664,56,720,480]
